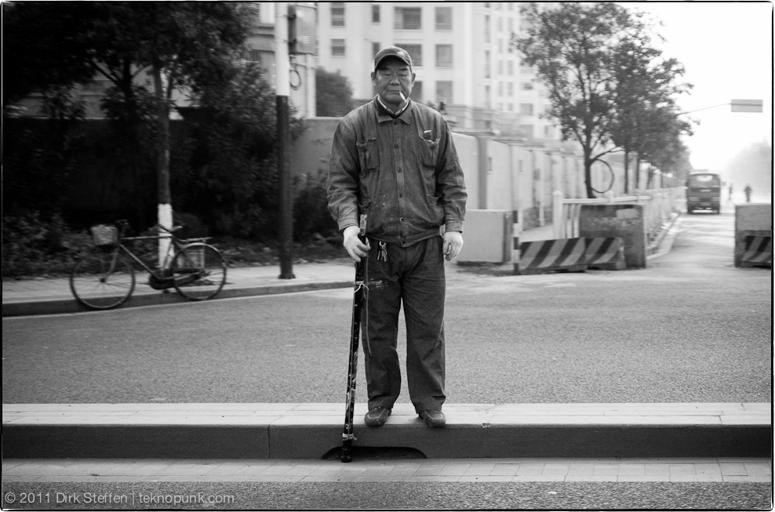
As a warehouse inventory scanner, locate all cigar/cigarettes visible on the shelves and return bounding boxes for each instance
[398,90,406,102]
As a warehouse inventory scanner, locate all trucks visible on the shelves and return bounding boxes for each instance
[684,168,721,215]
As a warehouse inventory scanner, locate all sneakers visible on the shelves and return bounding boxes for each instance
[419,409,446,428]
[365,407,391,427]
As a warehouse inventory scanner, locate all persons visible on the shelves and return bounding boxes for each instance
[744,182,752,204]
[326,46,468,429]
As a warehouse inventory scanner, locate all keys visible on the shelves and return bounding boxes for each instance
[377,241,388,263]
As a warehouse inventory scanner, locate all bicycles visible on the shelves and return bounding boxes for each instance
[68,215,228,311]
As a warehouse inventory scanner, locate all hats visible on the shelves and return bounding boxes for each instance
[375,46,411,70]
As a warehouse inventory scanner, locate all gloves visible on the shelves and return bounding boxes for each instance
[443,231,463,261]
[343,226,371,262]
